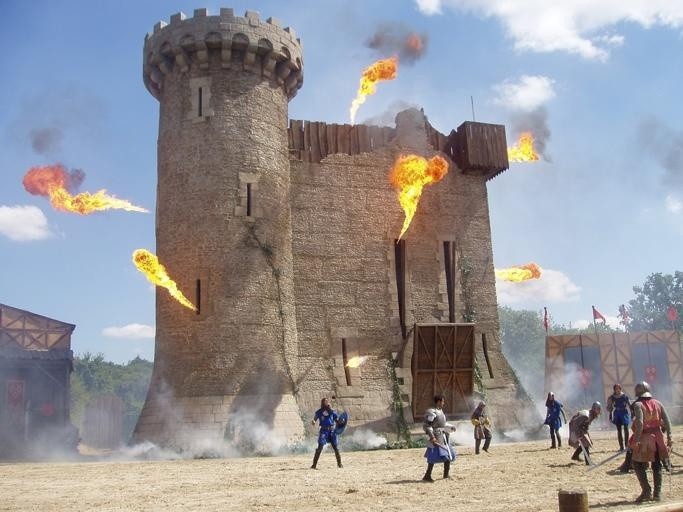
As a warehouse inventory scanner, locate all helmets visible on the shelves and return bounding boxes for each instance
[548,392,554,400]
[636,382,653,398]
[593,401,601,413]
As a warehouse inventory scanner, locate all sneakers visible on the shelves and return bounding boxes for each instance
[637,491,651,503]
[654,491,661,500]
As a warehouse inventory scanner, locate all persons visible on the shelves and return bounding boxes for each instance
[615,436,675,470]
[605,383,631,453]
[631,381,671,503]
[541,389,566,449]
[568,401,601,467]
[421,396,455,481]
[468,402,491,455]
[311,398,346,469]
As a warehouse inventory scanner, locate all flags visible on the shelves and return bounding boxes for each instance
[544,309,548,328]
[667,305,675,323]
[622,307,628,321]
[592,309,605,324]
